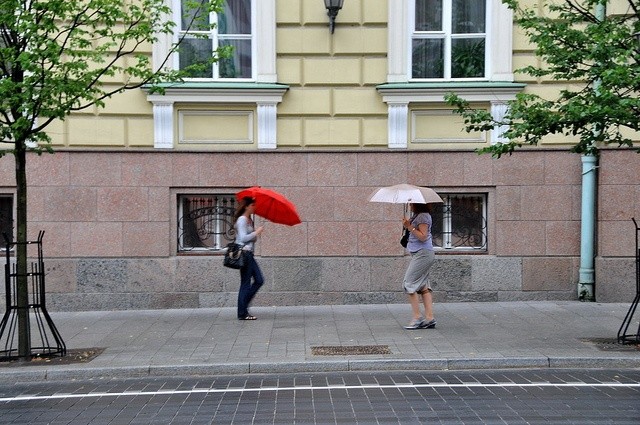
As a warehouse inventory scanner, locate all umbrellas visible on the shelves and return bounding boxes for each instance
[236,187,302,228]
[368,183,444,231]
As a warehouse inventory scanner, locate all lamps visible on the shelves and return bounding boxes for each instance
[324,0,344,35]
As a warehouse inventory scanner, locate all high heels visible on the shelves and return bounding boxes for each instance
[403,316,425,329]
[417,318,436,329]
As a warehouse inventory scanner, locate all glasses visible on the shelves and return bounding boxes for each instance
[248,197,255,203]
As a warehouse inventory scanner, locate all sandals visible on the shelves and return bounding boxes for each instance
[238,313,257,320]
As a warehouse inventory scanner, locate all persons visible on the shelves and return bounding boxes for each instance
[401,203,437,330]
[233,196,264,320]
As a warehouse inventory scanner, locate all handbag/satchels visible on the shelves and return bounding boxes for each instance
[223,221,255,269]
[400,213,418,248]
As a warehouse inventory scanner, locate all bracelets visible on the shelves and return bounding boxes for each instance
[411,228,416,233]
